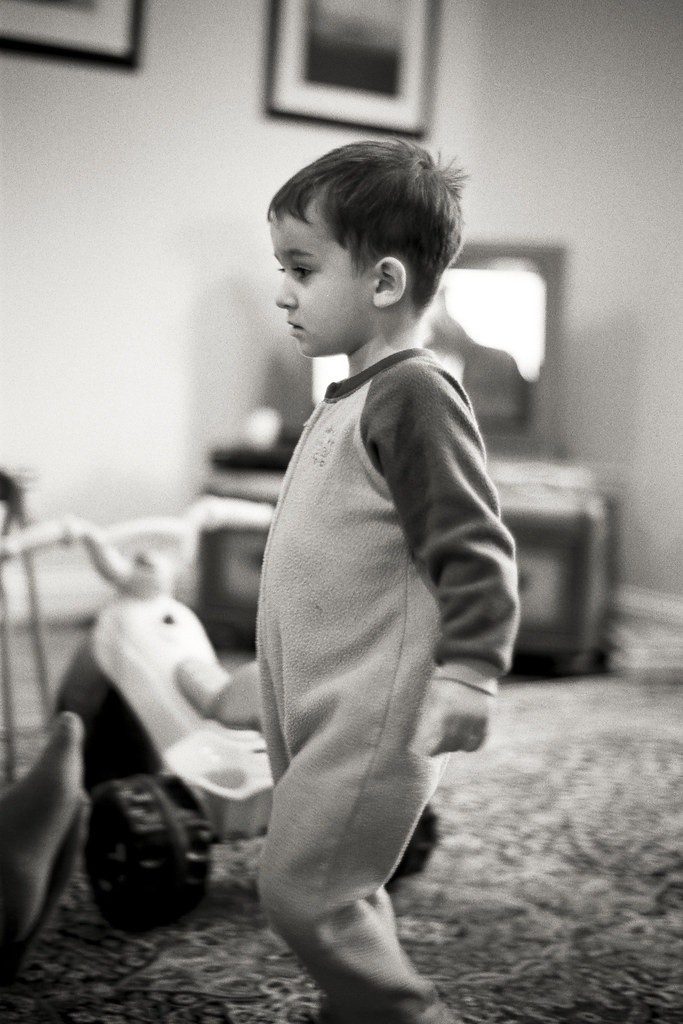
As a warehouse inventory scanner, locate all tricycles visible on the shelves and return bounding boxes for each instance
[0,492,442,932]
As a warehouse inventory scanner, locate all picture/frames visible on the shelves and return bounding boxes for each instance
[265,2,446,138]
[0,0,142,70]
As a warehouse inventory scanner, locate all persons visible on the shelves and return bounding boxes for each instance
[2,714,91,967]
[251,144,523,1024]
